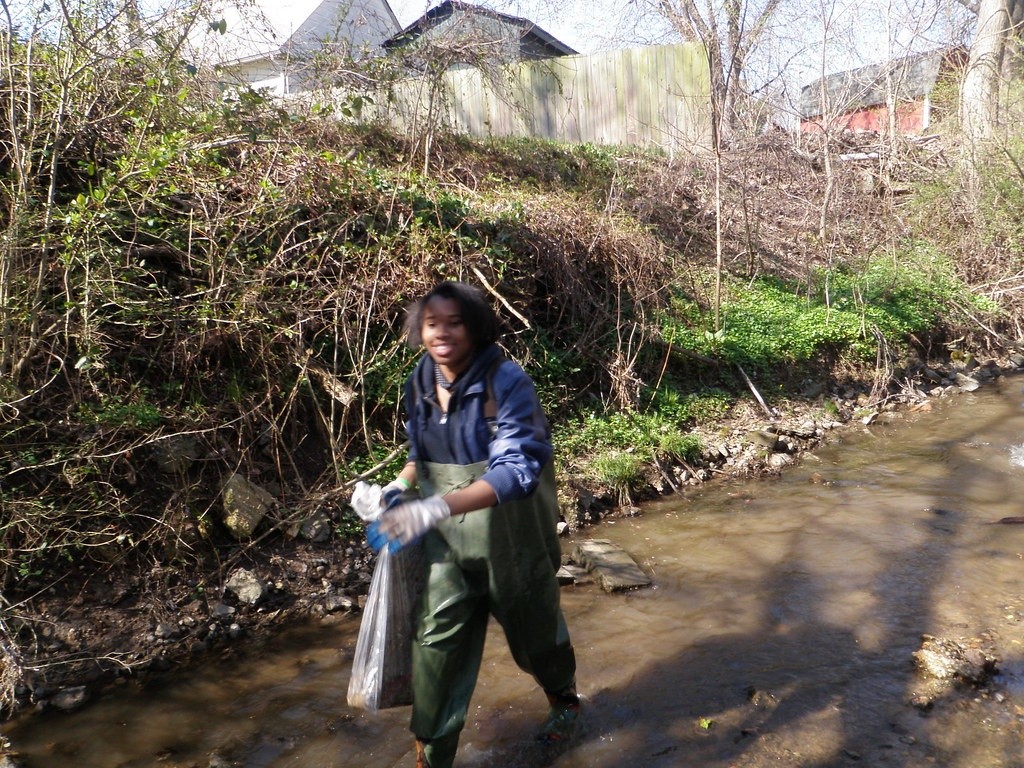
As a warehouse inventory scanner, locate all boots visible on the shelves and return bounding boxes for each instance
[535,677,577,747]
[415,732,460,768]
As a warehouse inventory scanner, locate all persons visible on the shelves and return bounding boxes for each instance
[363,283,581,768]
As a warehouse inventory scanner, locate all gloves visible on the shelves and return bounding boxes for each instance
[365,494,450,555]
[378,477,414,509]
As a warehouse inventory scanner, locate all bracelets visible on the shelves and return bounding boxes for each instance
[396,476,412,489]
